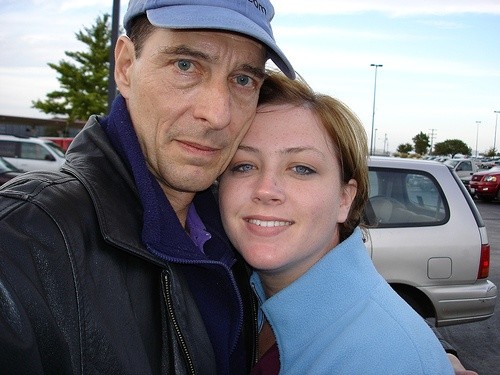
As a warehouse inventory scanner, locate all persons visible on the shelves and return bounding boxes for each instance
[0,0,479,375]
[217,68,457,375]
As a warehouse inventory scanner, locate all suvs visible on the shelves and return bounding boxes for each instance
[359,155,497,328]
[0,134,67,179]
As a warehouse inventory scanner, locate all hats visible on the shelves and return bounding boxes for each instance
[123,0,297,81]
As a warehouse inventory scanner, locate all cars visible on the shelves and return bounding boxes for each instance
[420,154,500,168]
[443,159,472,179]
[469,166,500,202]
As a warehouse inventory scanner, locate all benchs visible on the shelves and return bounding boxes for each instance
[391,208,440,222]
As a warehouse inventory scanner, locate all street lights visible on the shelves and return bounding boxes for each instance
[370,64,383,155]
